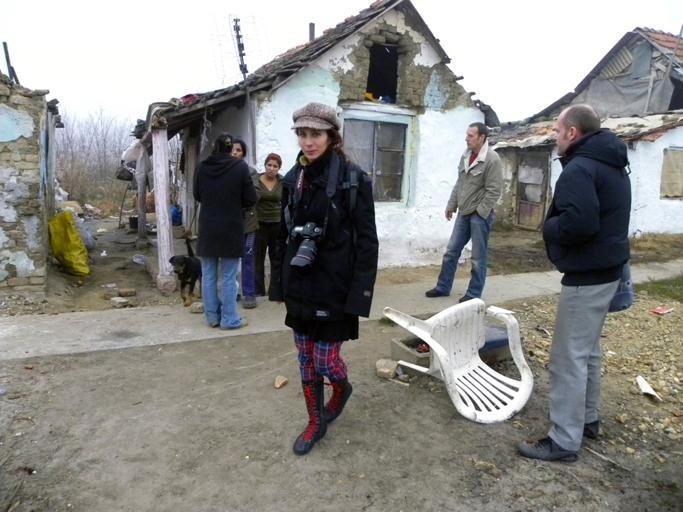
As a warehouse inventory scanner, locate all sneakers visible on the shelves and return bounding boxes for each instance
[583,422,599,439]
[425,288,444,297]
[518,437,576,461]
[244,297,256,308]
[459,295,473,303]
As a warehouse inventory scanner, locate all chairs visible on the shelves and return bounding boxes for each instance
[384,297,534,424]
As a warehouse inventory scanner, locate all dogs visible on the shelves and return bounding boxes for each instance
[168,238,202,307]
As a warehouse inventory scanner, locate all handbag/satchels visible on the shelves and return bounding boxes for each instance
[607,262,634,313]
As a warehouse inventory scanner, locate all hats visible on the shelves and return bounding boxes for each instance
[291,102,342,130]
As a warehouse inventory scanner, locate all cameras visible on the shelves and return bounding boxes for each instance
[290,222,327,275]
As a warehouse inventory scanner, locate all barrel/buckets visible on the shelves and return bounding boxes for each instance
[171,204,182,226]
[129,217,138,228]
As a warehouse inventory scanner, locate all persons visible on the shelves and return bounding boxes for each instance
[229,139,262,309]
[255,153,285,302]
[266,102,378,455]
[192,133,257,330]
[516,104,631,462]
[425,122,503,303]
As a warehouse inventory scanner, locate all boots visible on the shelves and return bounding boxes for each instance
[324,376,352,423]
[293,379,327,456]
